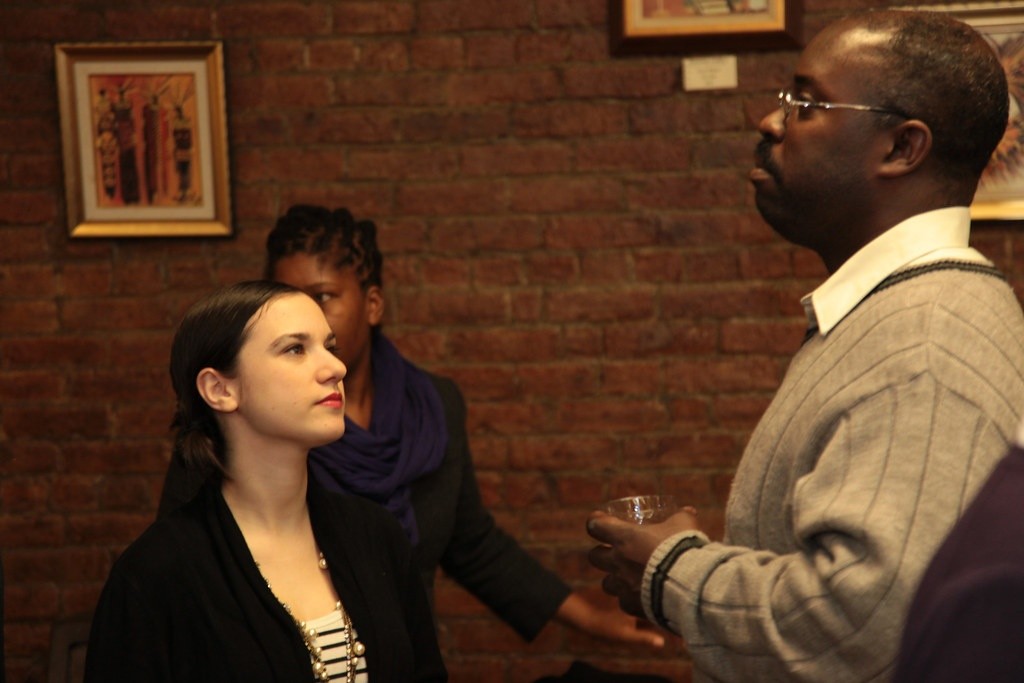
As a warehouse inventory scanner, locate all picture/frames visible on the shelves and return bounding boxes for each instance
[883,0,1024,221]
[607,1,802,57]
[52,40,237,238]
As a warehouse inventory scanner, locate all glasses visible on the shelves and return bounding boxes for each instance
[771,83,911,135]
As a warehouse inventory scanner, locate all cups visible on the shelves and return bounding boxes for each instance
[600,495,678,546]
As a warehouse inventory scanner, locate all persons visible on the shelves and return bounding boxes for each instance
[266,203,668,683]
[83,280,453,683]
[586,5,1023,683]
[896,443,1024,683]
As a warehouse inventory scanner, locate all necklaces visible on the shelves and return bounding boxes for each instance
[255,551,366,683]
